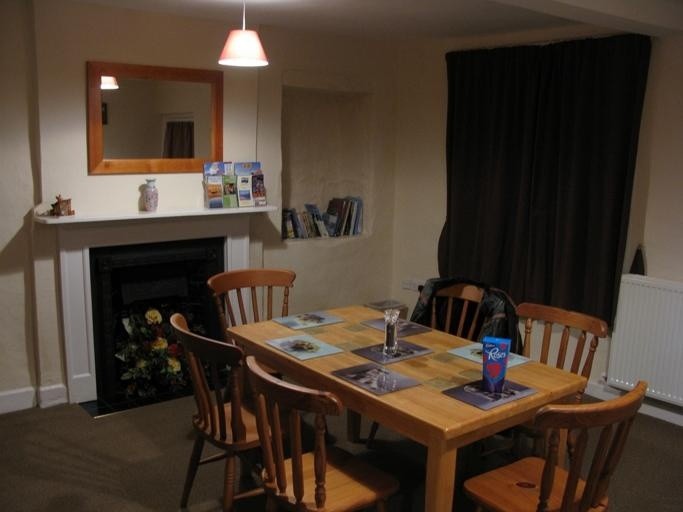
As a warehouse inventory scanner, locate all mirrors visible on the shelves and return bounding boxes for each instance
[87,62,224,176]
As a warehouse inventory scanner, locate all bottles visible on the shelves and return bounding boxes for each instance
[382,308,400,357]
[142,176,159,212]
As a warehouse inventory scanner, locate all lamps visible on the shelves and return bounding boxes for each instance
[218,0,269,67]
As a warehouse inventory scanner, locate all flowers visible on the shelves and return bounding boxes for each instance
[113,306,208,401]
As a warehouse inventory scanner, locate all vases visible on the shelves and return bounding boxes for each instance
[144,178,158,212]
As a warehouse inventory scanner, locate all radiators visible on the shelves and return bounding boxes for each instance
[601,273,683,407]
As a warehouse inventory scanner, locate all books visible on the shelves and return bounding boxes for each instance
[234,162,267,206]
[281,195,363,239]
[203,161,238,208]
